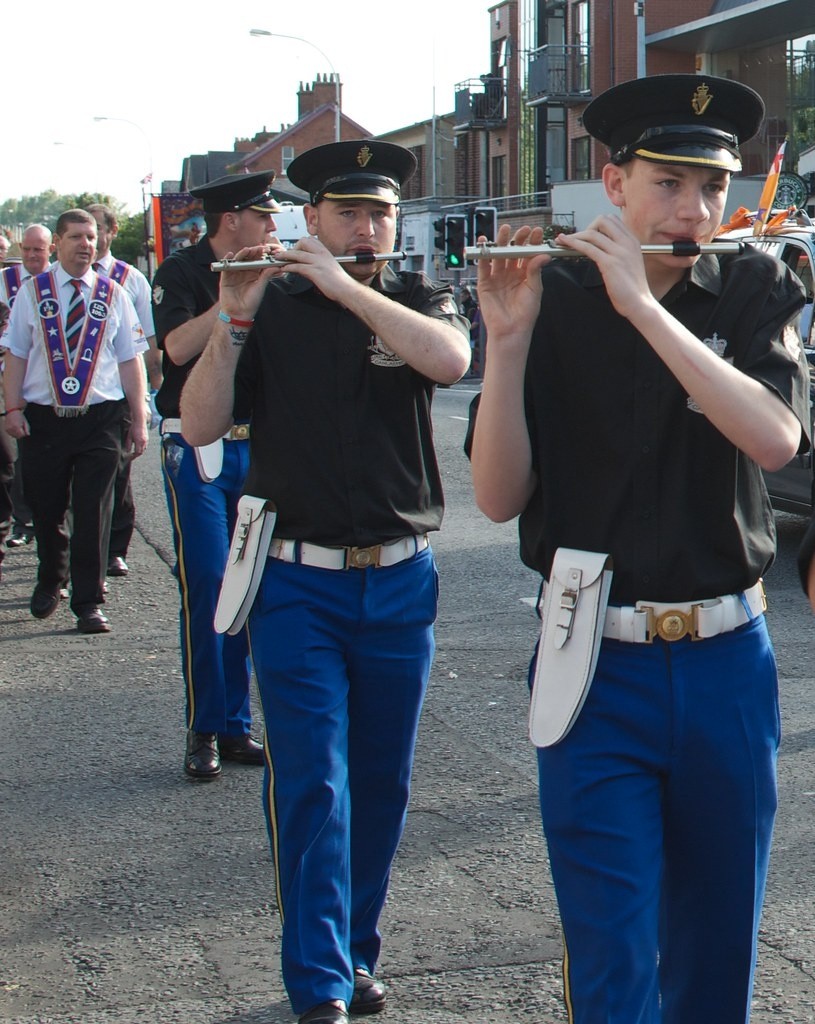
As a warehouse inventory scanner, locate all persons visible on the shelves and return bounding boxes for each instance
[179,141,473,1024]
[463,74,811,1024]
[0,204,162,633]
[151,170,286,778]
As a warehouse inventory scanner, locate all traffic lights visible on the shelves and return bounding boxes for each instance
[445,215,466,271]
[472,207,497,265]
[434,219,445,251]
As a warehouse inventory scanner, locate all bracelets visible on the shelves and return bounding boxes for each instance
[7,408,22,415]
[0,413,6,418]
[217,308,256,326]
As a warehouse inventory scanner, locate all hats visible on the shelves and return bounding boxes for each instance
[189,170,282,213]
[581,74,766,177]
[285,141,418,204]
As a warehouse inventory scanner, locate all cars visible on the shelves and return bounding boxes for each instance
[720,224,814,515]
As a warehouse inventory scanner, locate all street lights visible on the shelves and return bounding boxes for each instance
[251,29,340,144]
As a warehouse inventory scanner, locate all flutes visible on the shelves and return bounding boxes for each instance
[463,240,746,260]
[210,249,408,273]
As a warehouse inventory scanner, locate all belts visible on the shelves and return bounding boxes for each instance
[539,577,770,643]
[161,419,250,441]
[267,531,430,570]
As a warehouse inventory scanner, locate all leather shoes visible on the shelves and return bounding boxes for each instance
[76,603,112,633]
[5,534,31,548]
[28,584,60,619]
[107,555,129,576]
[217,737,266,766]
[298,999,349,1024]
[185,730,222,776]
[346,968,386,1014]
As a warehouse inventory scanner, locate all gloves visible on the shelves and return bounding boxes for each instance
[147,389,163,430]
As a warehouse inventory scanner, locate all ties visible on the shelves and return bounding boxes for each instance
[65,279,86,367]
